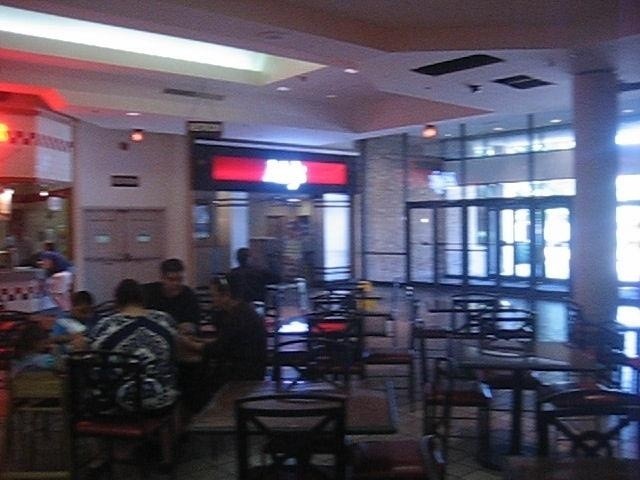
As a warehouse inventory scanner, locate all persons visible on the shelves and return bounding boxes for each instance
[12,325,71,414]
[54,288,93,352]
[178,275,267,394]
[31,241,77,318]
[86,279,182,417]
[140,259,199,336]
[225,245,280,301]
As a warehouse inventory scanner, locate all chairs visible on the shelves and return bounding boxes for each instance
[1,266,639,478]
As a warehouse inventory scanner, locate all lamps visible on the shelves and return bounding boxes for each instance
[131,128,144,143]
[422,125,437,138]
